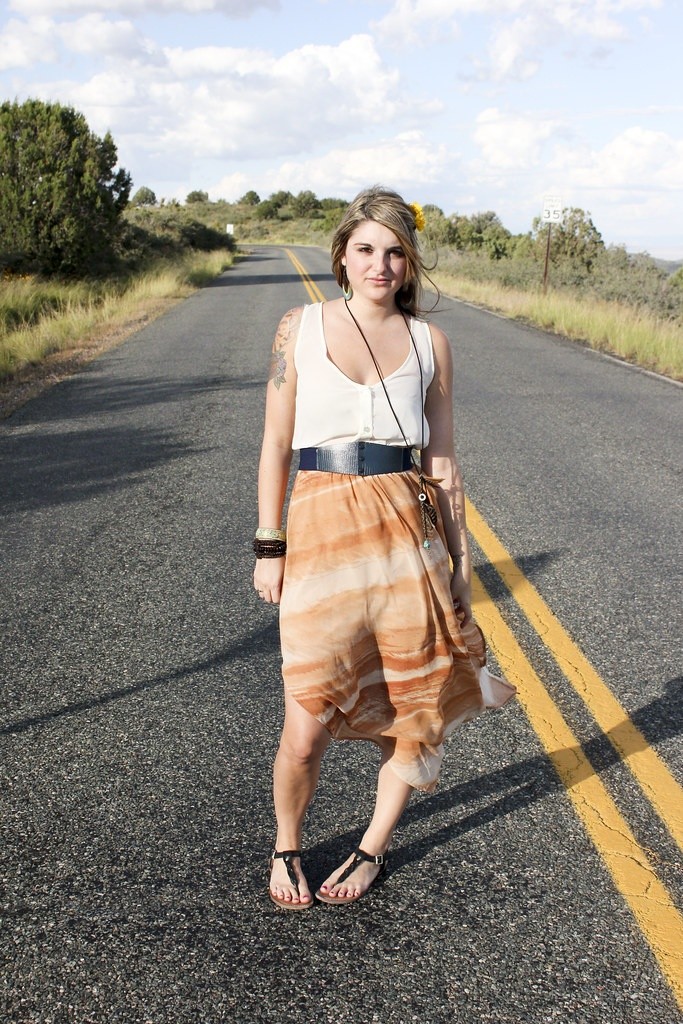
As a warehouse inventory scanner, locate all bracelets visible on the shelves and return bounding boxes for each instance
[250,527,289,560]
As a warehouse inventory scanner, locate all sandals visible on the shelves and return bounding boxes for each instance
[315,845,393,904]
[269,847,314,909]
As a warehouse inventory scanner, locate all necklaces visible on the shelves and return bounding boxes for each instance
[341,297,440,553]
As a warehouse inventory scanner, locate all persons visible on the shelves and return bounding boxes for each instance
[251,184,521,915]
